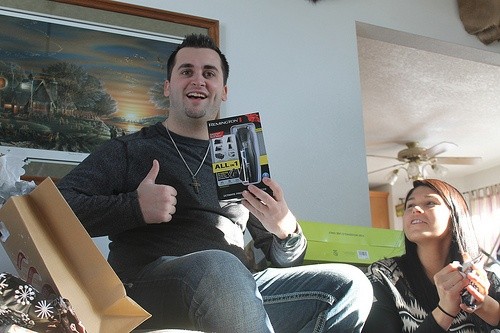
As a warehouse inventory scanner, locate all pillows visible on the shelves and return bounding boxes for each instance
[1,271,87,333]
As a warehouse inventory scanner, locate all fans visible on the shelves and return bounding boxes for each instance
[366,142,482,186]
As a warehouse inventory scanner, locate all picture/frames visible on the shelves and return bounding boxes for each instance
[0,0,219,185]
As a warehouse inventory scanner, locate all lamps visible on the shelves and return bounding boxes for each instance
[386,156,446,185]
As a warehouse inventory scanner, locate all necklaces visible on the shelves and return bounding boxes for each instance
[164,117,212,195]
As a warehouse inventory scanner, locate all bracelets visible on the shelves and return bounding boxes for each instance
[437,304,458,319]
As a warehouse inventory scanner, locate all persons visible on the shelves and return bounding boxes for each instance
[361,178,500,333]
[59,31,376,333]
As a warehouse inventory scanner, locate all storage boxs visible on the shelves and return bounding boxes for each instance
[207,113,273,204]
[296,219,409,266]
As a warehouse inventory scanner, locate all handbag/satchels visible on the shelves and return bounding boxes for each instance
[0,177,153,333]
